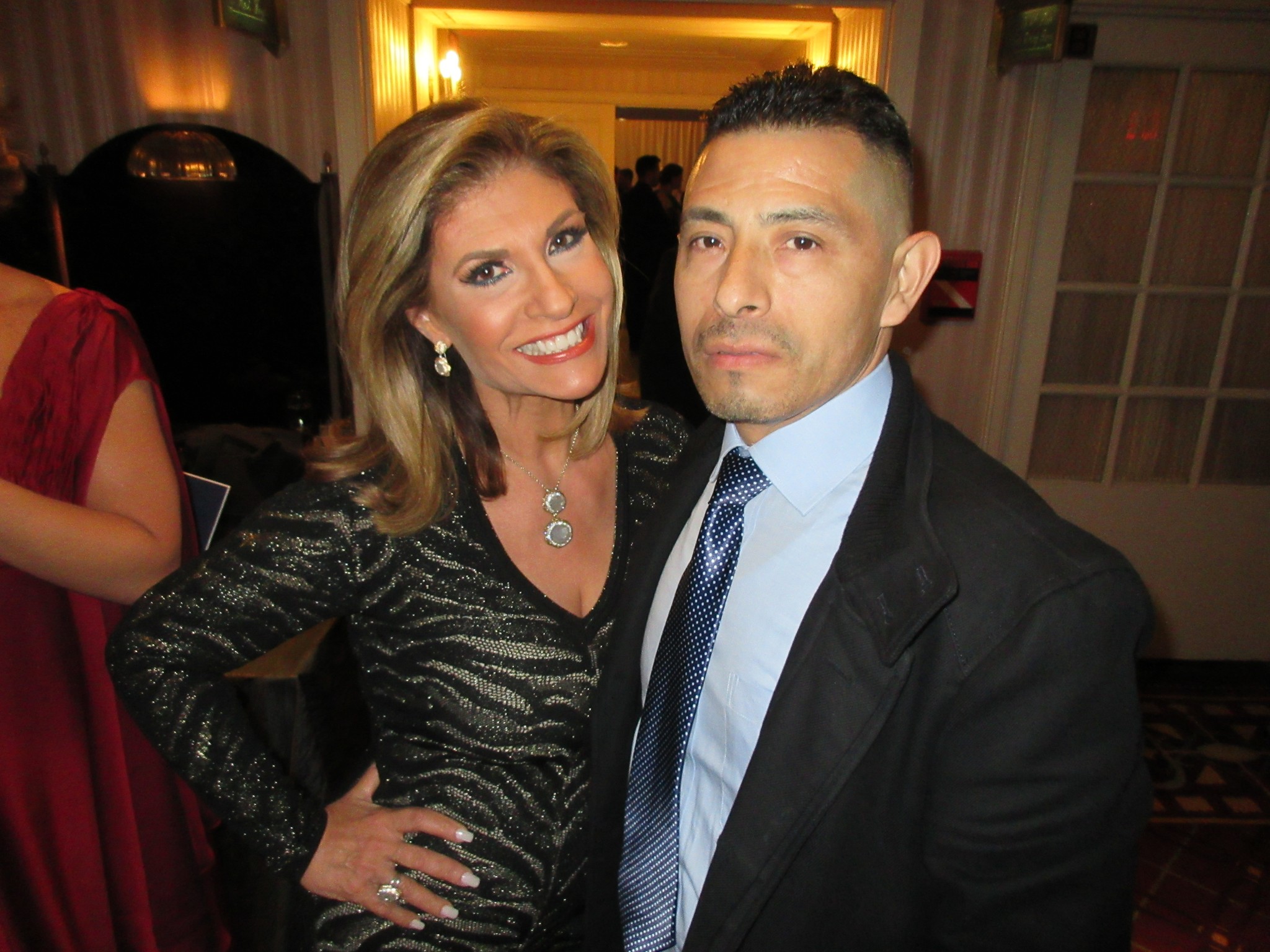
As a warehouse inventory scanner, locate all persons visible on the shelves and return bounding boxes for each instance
[606,154,707,418]
[1,142,249,952]
[107,97,699,951]
[585,54,1154,952]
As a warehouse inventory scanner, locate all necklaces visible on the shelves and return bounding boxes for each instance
[496,399,580,548]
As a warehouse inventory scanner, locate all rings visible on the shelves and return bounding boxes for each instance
[378,870,402,903]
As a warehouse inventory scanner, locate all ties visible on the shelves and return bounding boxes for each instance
[617,448,772,952]
